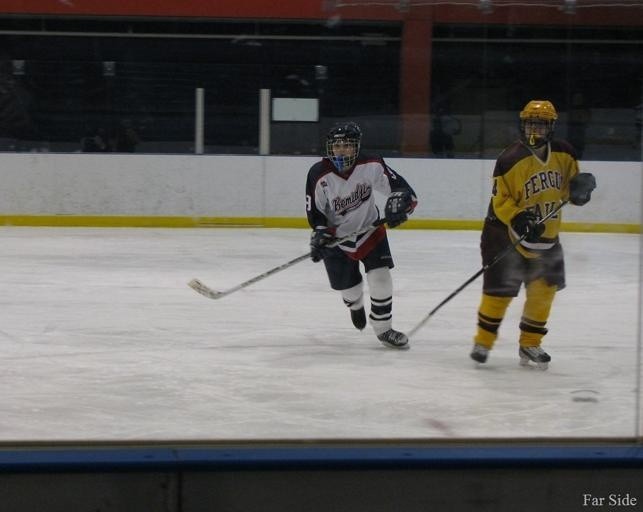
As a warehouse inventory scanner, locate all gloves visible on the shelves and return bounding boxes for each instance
[310,227,336,262]
[569,173,596,206]
[511,210,545,243]
[385,192,411,229]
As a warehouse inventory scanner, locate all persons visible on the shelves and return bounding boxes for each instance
[305,118,417,348]
[469,100,597,364]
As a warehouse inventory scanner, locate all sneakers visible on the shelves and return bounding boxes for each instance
[471,343,488,362]
[520,345,551,362]
[377,329,408,345]
[351,306,366,329]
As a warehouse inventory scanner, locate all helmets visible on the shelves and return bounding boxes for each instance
[326,121,362,172]
[520,100,562,149]
